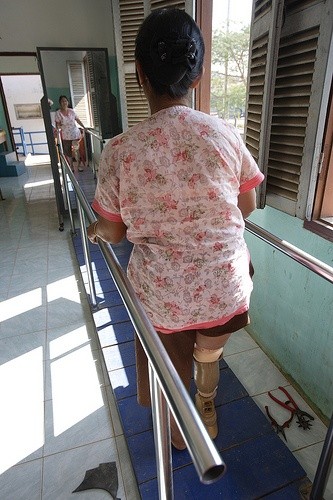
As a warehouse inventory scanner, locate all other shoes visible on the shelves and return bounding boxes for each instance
[194,391,219,439]
[71,166,74,173]
[78,166,84,172]
[170,411,187,450]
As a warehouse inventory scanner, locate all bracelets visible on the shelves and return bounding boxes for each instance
[93,221,99,237]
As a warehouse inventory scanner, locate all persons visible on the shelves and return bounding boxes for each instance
[55,95,92,172]
[85,7,265,453]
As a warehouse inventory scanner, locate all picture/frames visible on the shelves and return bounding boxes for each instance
[14,103,43,119]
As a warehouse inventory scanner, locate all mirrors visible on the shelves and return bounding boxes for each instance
[35,47,116,215]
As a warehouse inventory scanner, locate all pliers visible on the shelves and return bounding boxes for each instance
[268,386,315,430]
[265,405,294,443]
